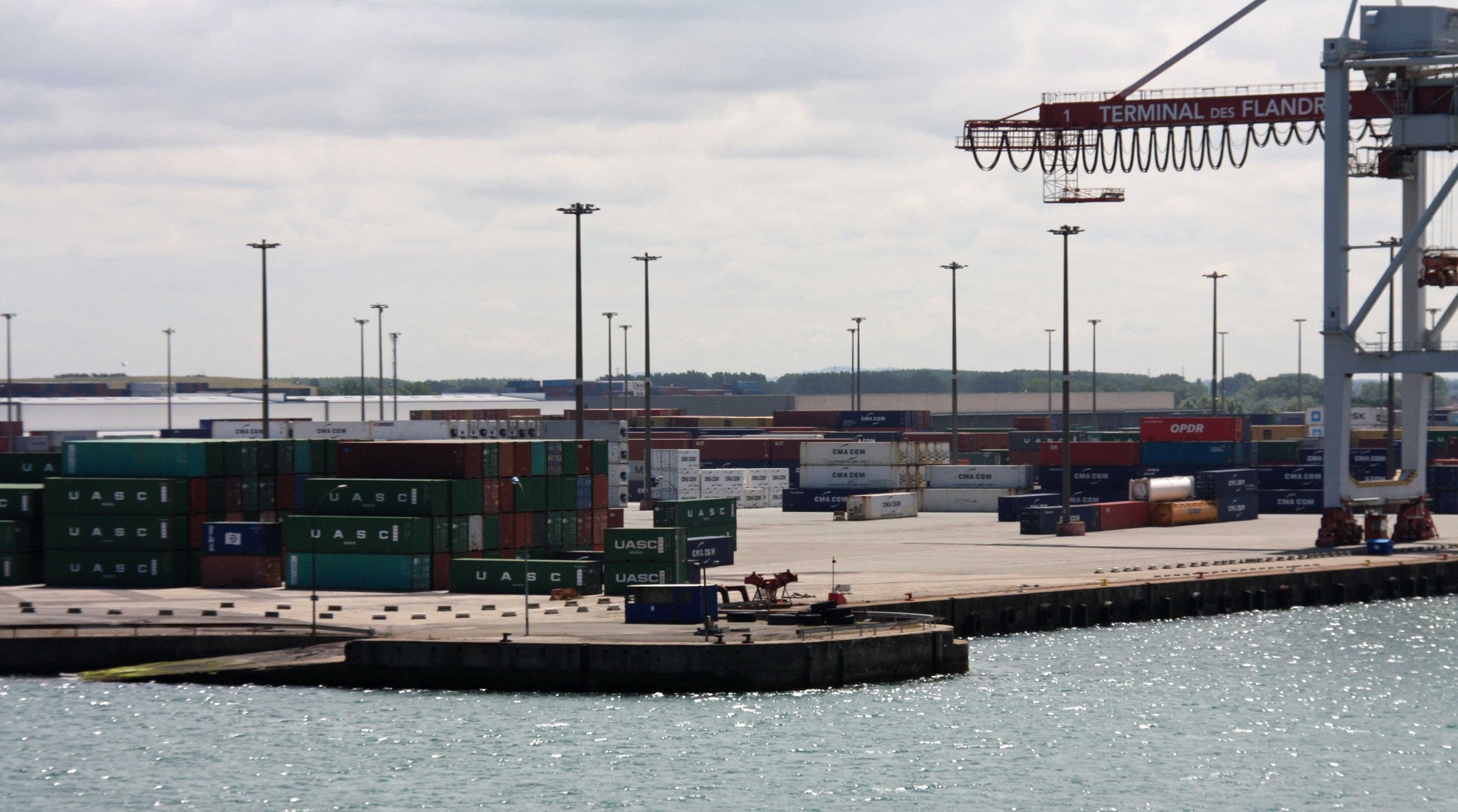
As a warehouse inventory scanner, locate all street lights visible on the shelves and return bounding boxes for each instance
[631,252,663,501]
[372,305,390,419]
[1376,236,1405,481]
[1202,273,1228,417]
[619,324,633,408]
[388,332,401,419]
[354,319,370,420]
[851,317,867,409]
[246,238,283,438]
[1,313,17,450]
[163,327,176,431]
[1219,330,1229,415]
[1048,224,1084,521]
[557,201,600,439]
[1088,319,1103,429]
[1044,328,1055,416]
[940,261,969,465]
[601,312,619,421]
[847,328,860,411]
[1294,319,1308,411]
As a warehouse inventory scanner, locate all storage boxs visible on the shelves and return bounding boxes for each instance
[0,380,1458,624]
